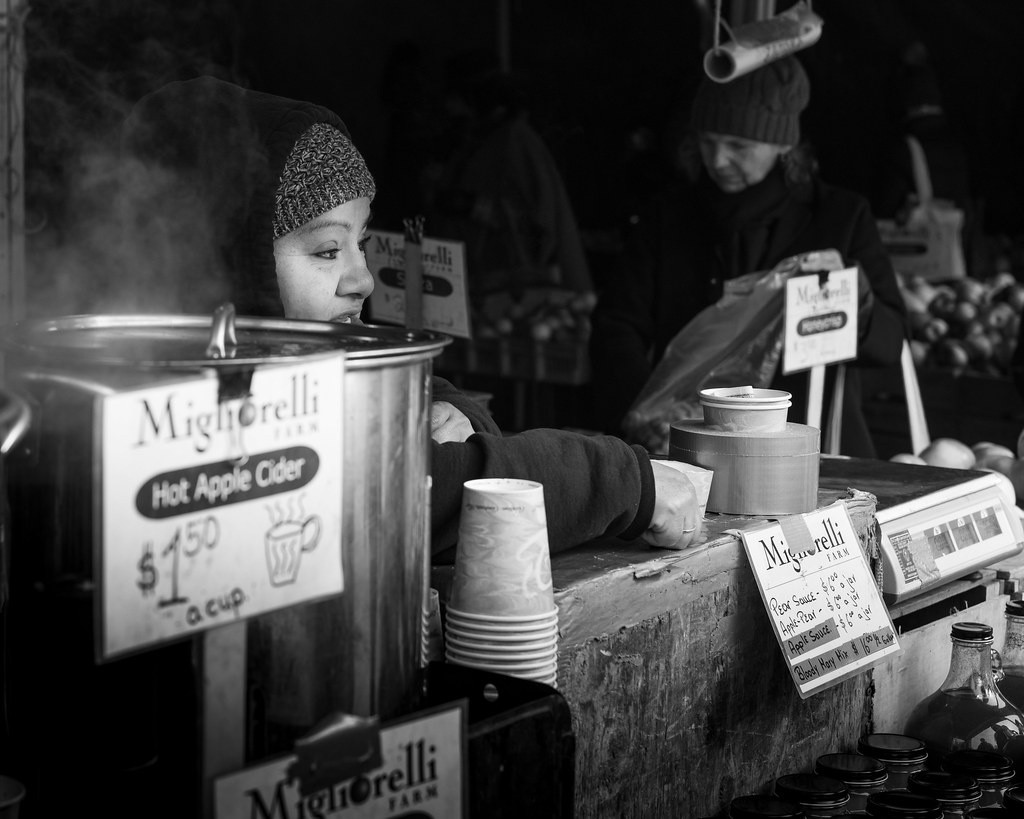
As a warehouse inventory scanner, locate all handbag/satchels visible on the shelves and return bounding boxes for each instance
[622,252,811,456]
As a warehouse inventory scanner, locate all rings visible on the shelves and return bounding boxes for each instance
[683,527,696,532]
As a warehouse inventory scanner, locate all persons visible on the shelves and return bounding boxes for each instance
[589,56,912,458]
[115,76,705,556]
[376,38,595,434]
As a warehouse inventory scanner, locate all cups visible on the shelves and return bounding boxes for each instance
[442,478,563,695]
[701,384,791,436]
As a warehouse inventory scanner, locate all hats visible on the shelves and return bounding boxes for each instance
[691,54,810,148]
[273,123,377,240]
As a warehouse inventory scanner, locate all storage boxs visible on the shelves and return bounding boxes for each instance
[881,220,967,285]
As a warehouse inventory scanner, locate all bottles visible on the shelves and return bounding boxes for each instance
[902,598,1024,792]
[711,732,1023,819]
